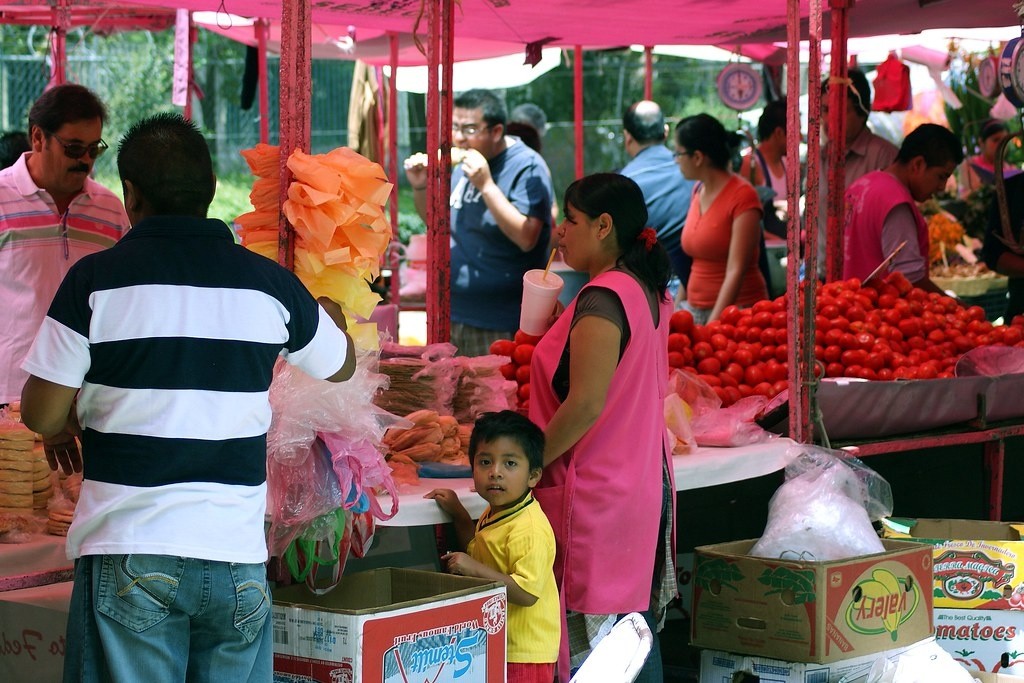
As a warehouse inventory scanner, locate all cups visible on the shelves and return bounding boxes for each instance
[520,268,565,337]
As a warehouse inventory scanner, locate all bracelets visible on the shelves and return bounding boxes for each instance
[414,185,426,191]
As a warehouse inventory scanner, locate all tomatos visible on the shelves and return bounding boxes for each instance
[799,270,1024,381]
[490,330,541,408]
[668,293,789,408]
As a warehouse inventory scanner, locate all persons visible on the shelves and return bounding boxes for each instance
[422,411,560,683]
[404,87,555,358]
[502,68,1023,325]
[529,171,677,683]
[0,85,133,414]
[0,129,31,172]
[16,114,355,683]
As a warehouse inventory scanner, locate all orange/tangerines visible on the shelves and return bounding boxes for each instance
[929,213,964,262]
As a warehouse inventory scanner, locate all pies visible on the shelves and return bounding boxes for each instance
[0,401,79,536]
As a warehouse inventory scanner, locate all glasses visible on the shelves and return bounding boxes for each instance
[452,125,492,135]
[54,133,108,159]
[672,151,688,158]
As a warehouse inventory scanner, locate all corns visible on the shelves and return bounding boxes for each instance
[421,147,465,167]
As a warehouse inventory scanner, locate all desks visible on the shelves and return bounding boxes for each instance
[0,422,1024,594]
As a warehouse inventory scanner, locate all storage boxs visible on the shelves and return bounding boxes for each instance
[0,581,74,683]
[677,515,1024,683]
[269,566,507,683]
